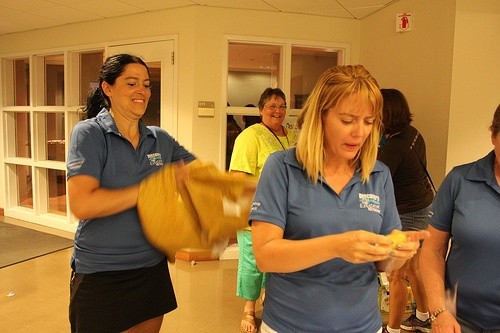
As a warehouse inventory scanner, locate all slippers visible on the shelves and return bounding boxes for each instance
[240,303,257,333]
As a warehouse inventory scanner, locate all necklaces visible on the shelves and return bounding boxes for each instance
[386,133,393,141]
[261,121,289,150]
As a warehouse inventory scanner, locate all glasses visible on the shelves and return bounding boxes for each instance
[264,103,286,110]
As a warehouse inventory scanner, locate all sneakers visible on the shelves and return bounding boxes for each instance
[382,326,390,333]
[400,312,432,333]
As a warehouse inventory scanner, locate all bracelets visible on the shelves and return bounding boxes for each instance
[434,307,446,317]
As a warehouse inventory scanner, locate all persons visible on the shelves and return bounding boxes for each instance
[418,105,500,333]
[229,88,297,333]
[247,65,432,333]
[377,88,436,333]
[67,54,202,333]
[227,104,242,173]
[243,104,262,129]
